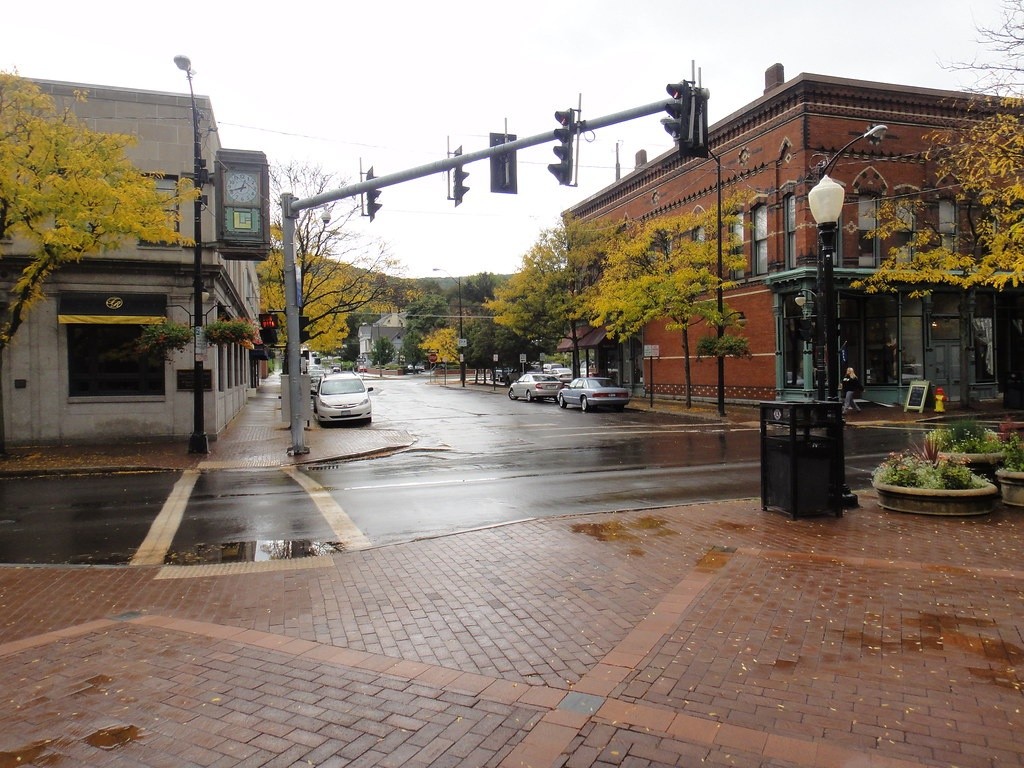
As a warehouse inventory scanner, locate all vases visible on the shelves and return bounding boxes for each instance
[995,470,1024,507]
[938,452,1003,486]
[871,481,999,515]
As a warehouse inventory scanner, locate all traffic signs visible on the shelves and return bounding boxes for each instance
[357,358,366,362]
[520,354,527,363]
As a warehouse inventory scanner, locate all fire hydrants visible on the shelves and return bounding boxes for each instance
[933,388,949,413]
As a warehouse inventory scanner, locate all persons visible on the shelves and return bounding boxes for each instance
[841,367,864,415]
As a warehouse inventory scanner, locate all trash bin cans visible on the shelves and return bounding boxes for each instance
[759,401,845,521]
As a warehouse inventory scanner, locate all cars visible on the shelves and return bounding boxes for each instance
[557,377,631,411]
[310,373,373,425]
[405,365,426,374]
[508,372,564,402]
[358,366,368,372]
[330,362,340,372]
[491,363,586,381]
[307,365,327,393]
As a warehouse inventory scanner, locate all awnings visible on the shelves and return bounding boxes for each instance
[557,321,620,349]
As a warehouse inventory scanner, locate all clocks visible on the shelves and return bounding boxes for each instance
[226,173,258,203]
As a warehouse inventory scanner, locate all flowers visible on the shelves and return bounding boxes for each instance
[203,314,263,348]
[133,315,194,366]
[872,413,1024,490]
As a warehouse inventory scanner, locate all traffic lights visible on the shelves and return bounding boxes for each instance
[454,146,470,206]
[660,80,687,144]
[259,314,278,344]
[299,316,310,343]
[367,166,382,221]
[548,108,572,185]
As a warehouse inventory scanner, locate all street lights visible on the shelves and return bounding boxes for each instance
[362,322,382,377]
[807,174,859,510]
[173,55,211,454]
[660,116,748,414]
[795,125,887,400]
[433,267,465,381]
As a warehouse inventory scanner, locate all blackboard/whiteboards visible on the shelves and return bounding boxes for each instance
[907,385,925,407]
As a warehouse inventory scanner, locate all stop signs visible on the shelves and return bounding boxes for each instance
[429,354,437,363]
